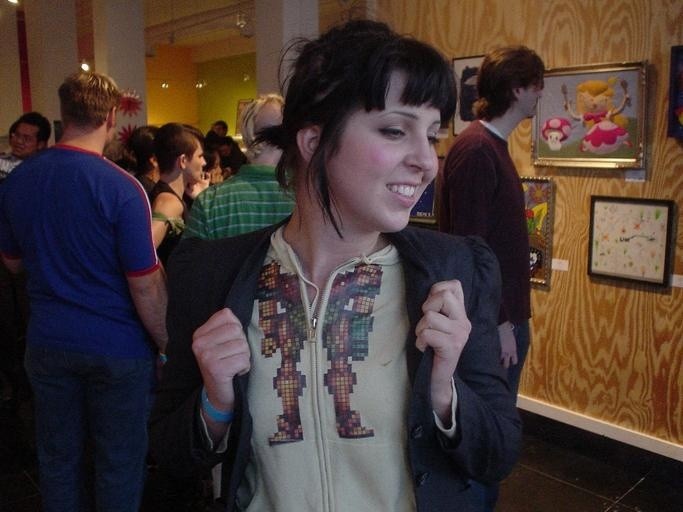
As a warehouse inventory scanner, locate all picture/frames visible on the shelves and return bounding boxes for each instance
[586,195,675,288]
[530,60,648,171]
[450,54,486,137]
[520,174,555,286]
[666,44,683,138]
[408,155,445,224]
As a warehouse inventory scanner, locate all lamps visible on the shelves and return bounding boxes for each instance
[235,13,247,29]
[191,79,208,89]
[159,79,169,89]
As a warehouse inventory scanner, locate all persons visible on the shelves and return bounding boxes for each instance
[104,94,288,510]
[154,14,526,510]
[435,41,547,511]
[0,111,51,179]
[0,71,172,511]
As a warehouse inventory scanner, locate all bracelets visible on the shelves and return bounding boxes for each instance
[496,322,515,332]
